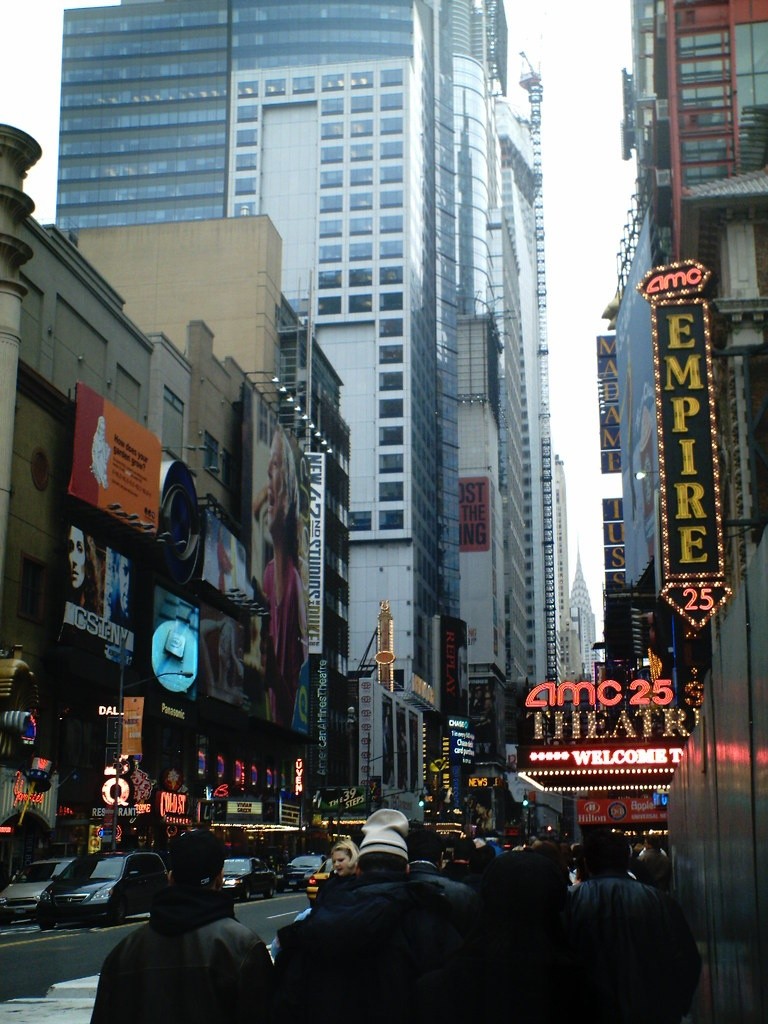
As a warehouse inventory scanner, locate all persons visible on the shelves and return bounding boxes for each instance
[274,808,701,1024]
[66,430,308,722]
[91,828,275,1024]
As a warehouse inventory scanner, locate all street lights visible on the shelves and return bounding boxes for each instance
[523,799,531,847]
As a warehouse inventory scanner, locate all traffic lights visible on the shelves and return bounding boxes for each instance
[419,795,425,808]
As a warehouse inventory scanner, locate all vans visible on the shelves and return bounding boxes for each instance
[284,854,328,894]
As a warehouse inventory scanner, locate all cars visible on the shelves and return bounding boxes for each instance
[38,851,171,930]
[0,855,87,927]
[308,859,335,906]
[223,856,278,902]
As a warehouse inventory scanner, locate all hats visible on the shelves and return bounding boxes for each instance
[167,828,224,886]
[478,847,569,908]
[357,809,410,860]
